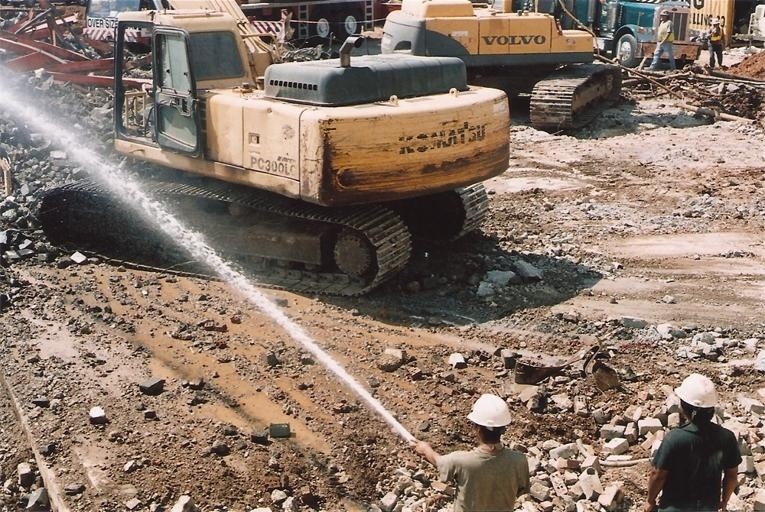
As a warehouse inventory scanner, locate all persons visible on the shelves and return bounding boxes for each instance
[643,372,743,512]
[641,9,677,72]
[410,391,531,512]
[705,21,727,69]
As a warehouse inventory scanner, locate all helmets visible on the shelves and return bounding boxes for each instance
[467,394,512,428]
[660,11,669,15]
[710,19,720,24]
[675,374,717,408]
